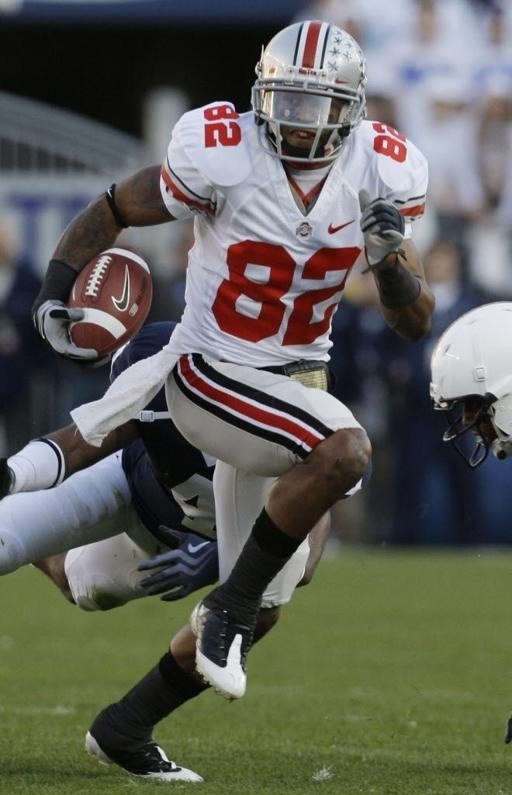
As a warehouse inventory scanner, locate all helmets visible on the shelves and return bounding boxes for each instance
[257,20,367,130]
[428,300,510,464]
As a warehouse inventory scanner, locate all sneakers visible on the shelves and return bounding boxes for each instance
[83,700,209,785]
[190,582,261,704]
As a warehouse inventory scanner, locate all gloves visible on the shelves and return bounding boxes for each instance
[136,521,216,604]
[355,187,407,271]
[29,294,117,370]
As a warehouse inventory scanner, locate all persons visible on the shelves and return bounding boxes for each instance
[25,18,439,787]
[0,320,336,613]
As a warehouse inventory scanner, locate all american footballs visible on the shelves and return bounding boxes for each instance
[66,246,156,355]
